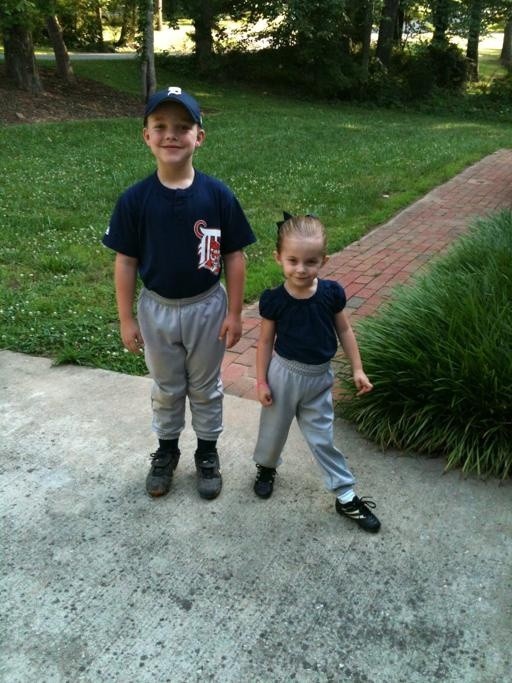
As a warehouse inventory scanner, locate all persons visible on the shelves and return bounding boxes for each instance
[244,209,384,534]
[96,83,259,502]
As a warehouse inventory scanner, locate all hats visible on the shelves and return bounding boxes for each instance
[144,86,201,129]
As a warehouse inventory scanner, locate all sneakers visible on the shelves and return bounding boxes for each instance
[194,448,223,500]
[146,446,181,497]
[336,494,381,533]
[254,463,276,499]
